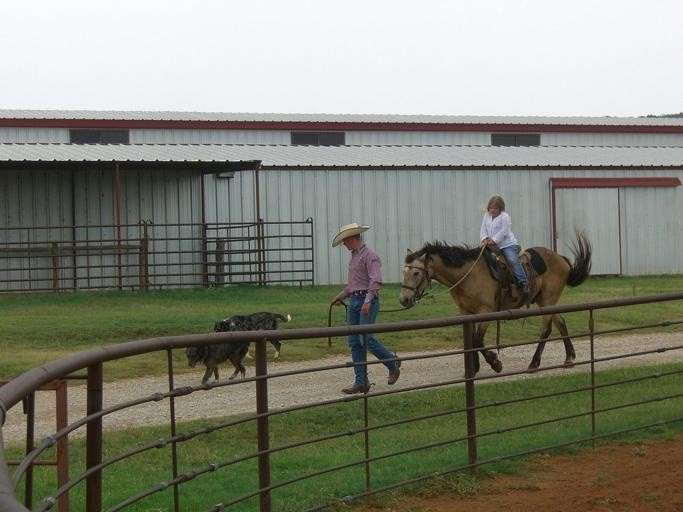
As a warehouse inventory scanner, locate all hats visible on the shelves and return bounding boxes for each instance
[332,223,371,247]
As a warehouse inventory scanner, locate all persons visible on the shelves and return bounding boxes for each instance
[480,195,529,294]
[331,223,401,395]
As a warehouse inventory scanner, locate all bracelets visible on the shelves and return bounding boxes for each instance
[365,298,370,304]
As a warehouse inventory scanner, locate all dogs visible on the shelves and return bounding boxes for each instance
[186,342,251,385]
[213,311,292,360]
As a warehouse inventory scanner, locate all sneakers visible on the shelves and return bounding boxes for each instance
[342,383,370,394]
[388,361,402,384]
[523,285,529,293]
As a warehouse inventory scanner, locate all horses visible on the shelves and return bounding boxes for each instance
[399,226,593,374]
